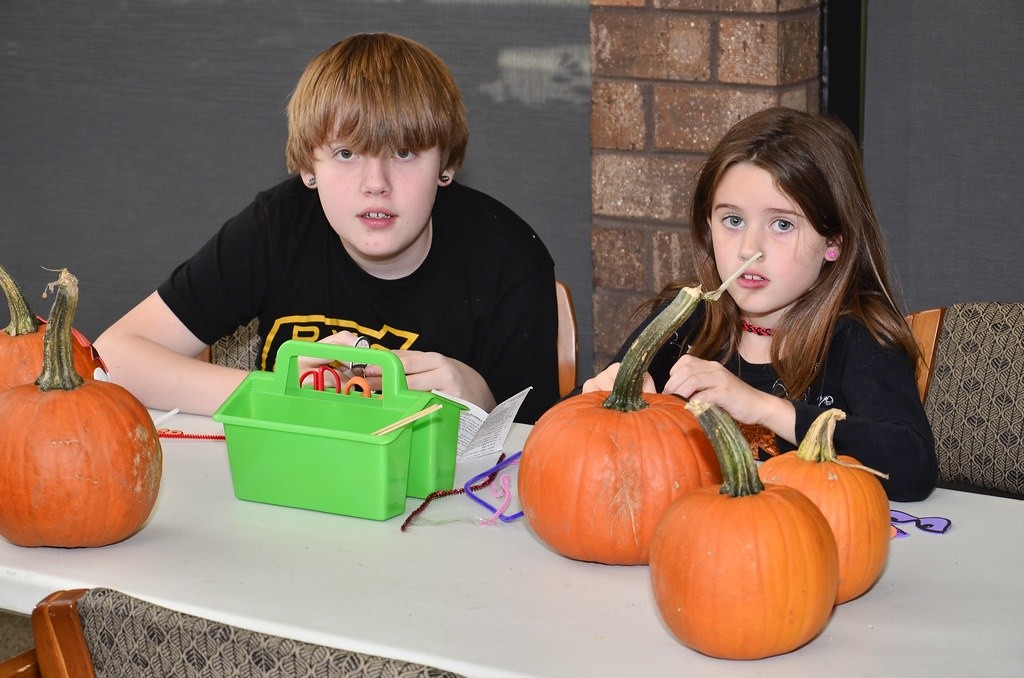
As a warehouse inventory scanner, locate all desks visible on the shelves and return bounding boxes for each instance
[2,390,1023,677]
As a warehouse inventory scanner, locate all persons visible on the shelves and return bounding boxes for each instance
[564,105,940,504]
[90,31,563,426]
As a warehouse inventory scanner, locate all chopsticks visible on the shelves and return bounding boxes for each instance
[370,403,443,437]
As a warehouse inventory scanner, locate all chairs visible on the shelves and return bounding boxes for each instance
[894,300,1024,501]
[30,588,462,678]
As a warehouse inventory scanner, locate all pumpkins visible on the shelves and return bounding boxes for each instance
[0,265,162,548]
[756,409,891,606]
[517,284,723,567]
[649,405,840,661]
